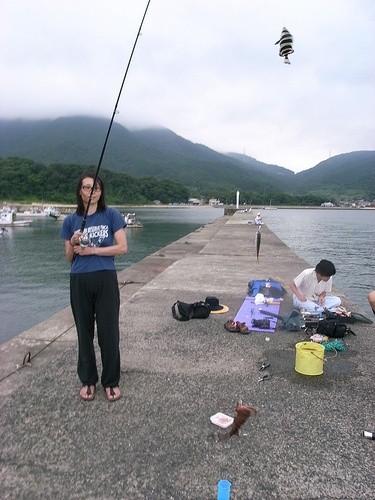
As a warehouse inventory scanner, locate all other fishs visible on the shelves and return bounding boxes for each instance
[219,402,257,445]
[274,26,295,65]
[255,229,262,264]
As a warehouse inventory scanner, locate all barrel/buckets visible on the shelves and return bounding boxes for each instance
[293,341,326,375]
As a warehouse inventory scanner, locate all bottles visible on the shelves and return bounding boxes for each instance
[217,479,230,500]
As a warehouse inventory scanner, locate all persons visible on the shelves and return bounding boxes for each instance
[255,213,263,224]
[60,175,129,402]
[290,259,341,312]
[368,291,375,316]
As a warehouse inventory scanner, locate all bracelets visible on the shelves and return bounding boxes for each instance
[69,241,74,247]
[94,248,95,255]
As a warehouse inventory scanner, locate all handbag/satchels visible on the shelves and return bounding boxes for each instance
[283,310,301,330]
[171,299,213,321]
[248,279,288,298]
[316,320,357,339]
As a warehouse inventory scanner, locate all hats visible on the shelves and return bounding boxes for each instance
[202,295,224,311]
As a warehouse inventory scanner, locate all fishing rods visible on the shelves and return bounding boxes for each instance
[70,0,150,263]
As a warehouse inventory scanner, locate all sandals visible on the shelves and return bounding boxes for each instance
[79,383,96,401]
[224,320,240,332]
[236,321,250,334]
[104,386,121,401]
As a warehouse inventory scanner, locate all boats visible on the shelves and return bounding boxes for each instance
[122,212,144,228]
[0,205,77,227]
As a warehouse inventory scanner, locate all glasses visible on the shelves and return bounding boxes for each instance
[82,186,101,193]
